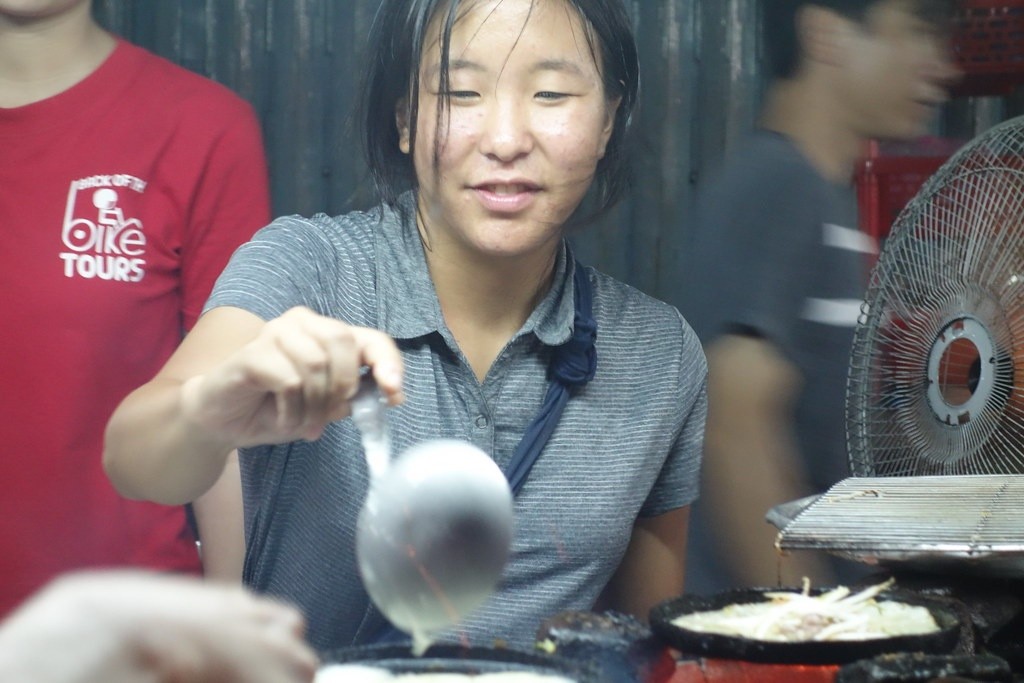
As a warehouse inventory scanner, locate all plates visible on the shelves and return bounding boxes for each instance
[767,495,1024,578]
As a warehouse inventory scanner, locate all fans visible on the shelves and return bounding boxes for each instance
[843,114,1024,477]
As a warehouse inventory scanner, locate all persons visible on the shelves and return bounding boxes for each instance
[632,1,974,617]
[1,0,279,627]
[0,555,321,683]
[101,2,714,682]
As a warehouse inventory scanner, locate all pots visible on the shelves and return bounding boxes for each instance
[647,586,970,683]
